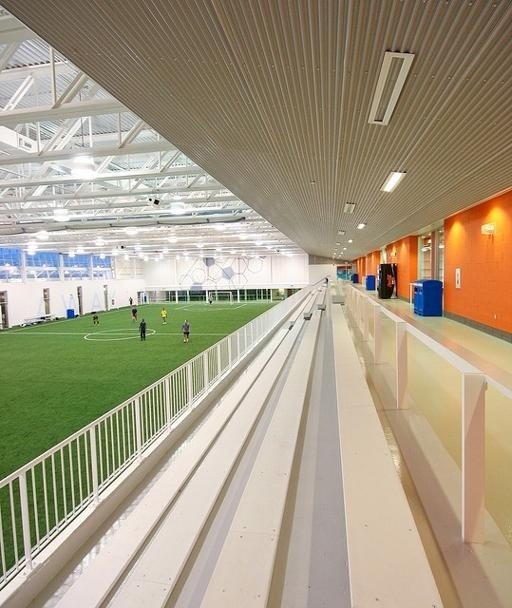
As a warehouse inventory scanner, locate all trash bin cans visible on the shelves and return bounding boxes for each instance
[366,275,375,291]
[412,279,443,316]
[353,274,358,283]
[67,309,74,319]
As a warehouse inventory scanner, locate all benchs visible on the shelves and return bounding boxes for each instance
[42,281,447,606]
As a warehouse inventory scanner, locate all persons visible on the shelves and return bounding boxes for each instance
[209,296,213,305]
[160,308,168,324]
[181,319,190,343]
[93,314,100,328]
[131,306,137,323]
[138,319,146,341]
[129,297,132,306]
